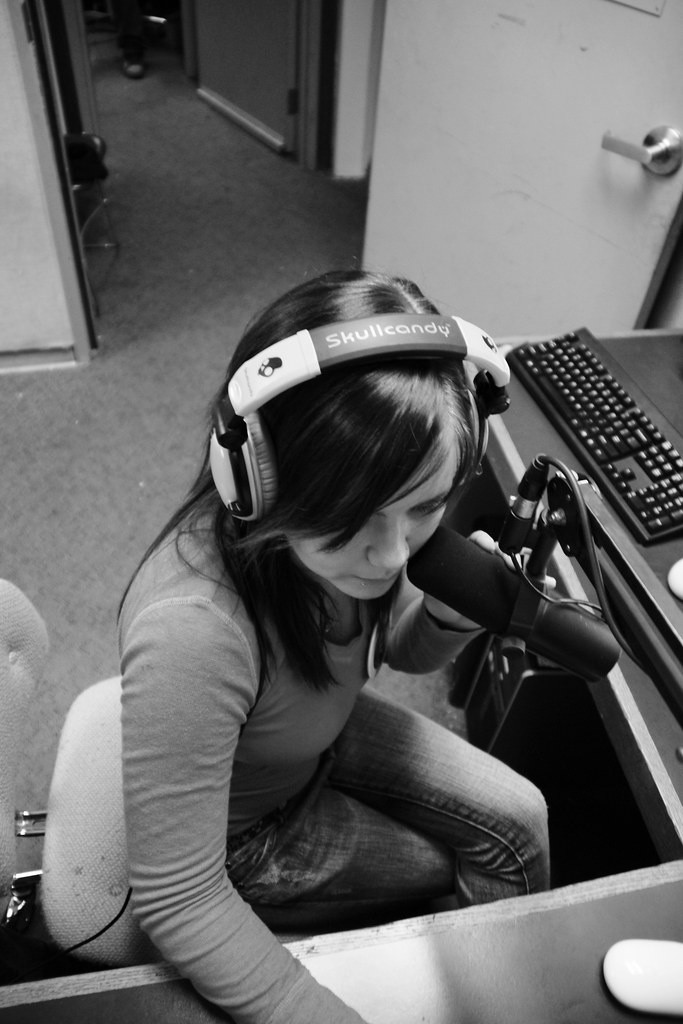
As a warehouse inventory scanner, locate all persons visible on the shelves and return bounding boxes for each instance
[111,0,176,79]
[120,270,556,1024]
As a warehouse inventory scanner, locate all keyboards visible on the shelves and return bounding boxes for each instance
[503,332,683,546]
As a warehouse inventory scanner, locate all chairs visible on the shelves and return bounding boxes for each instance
[0,578,156,965]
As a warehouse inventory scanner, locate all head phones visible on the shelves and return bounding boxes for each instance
[210,315,511,523]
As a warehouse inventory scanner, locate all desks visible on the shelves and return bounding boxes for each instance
[0,329,683,1024]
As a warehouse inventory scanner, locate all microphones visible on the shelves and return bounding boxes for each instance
[499,452,550,556]
[407,526,622,682]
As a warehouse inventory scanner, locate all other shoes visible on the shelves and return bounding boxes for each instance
[121,49,147,78]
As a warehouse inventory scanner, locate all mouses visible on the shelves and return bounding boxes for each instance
[667,558,683,601]
[601,940,683,1018]
